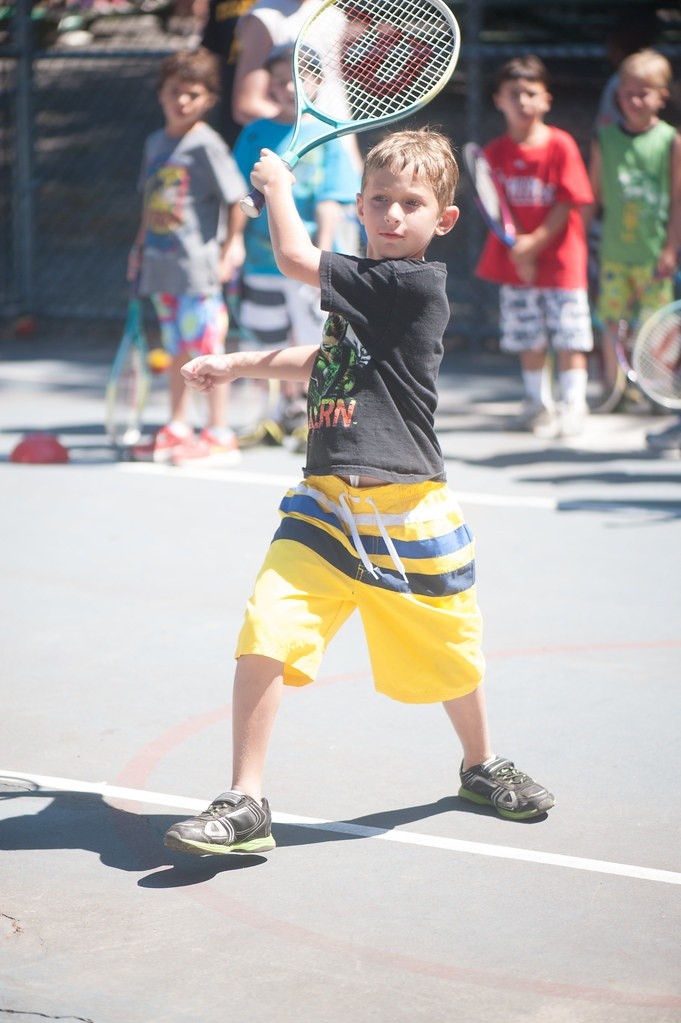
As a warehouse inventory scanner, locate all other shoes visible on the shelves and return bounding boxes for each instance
[556,402,594,440]
[523,407,561,438]
[646,423,681,451]
[128,425,191,463]
[172,425,241,463]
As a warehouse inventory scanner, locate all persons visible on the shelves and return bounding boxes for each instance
[164,128,555,859]
[230,43,359,455]
[231,0,374,458]
[591,48,680,422]
[475,53,596,440]
[125,47,252,469]
[587,3,680,350]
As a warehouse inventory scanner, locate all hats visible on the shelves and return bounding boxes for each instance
[263,42,323,70]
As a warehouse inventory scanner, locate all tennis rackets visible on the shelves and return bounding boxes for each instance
[614,269,681,412]
[462,140,518,249]
[104,267,150,450]
[237,2,461,220]
[219,272,271,450]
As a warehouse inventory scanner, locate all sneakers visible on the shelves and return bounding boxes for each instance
[458,755,554,820]
[165,791,275,854]
